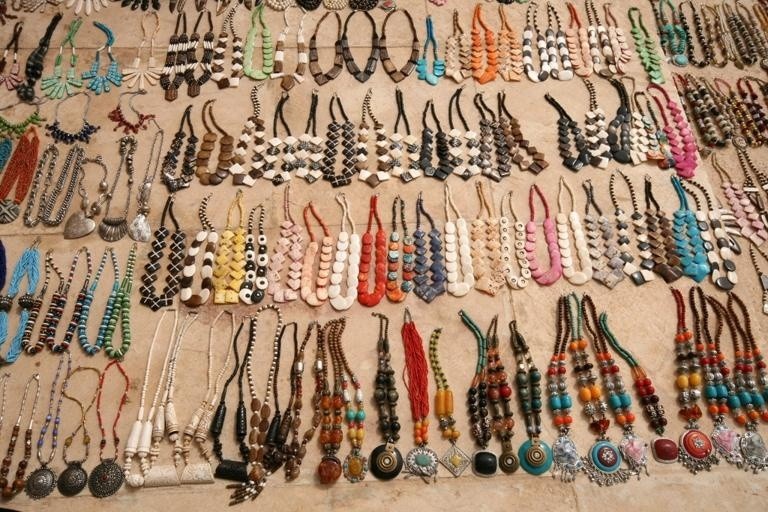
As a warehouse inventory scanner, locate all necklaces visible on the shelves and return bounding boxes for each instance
[620,74,649,166]
[121,308,179,488]
[415,15,445,85]
[239,203,268,306]
[469,3,499,85]
[699,75,748,149]
[413,190,447,303]
[722,0,759,66]
[180,193,219,308]
[387,193,415,304]
[138,194,187,312]
[689,285,745,471]
[605,78,632,165]
[195,99,235,186]
[78,247,120,356]
[444,85,482,181]
[598,308,679,465]
[671,69,711,159]
[524,182,563,287]
[647,82,699,179]
[161,12,188,103]
[357,85,392,190]
[0,128,41,224]
[678,0,712,67]
[669,174,709,283]
[44,145,85,227]
[145,310,200,486]
[387,85,421,184]
[1,373,41,500]
[648,0,673,66]
[445,8,472,84]
[544,93,593,172]
[684,71,732,148]
[228,80,268,188]
[56,366,101,497]
[495,89,551,177]
[87,361,129,498]
[81,20,123,96]
[626,7,666,86]
[500,189,532,291]
[357,193,387,307]
[682,175,738,291]
[564,1,592,78]
[522,0,549,83]
[0,19,22,91]
[377,8,420,83]
[342,9,380,83]
[40,15,84,100]
[261,93,295,189]
[418,97,453,183]
[580,176,626,291]
[270,4,308,92]
[243,1,274,81]
[659,1,689,68]
[322,93,359,188]
[64,154,111,240]
[442,181,474,298]
[97,134,138,242]
[20,249,65,356]
[604,0,632,76]
[470,178,508,296]
[284,319,322,483]
[545,2,573,82]
[399,305,440,484]
[160,105,199,194]
[459,308,498,478]
[609,166,656,287]
[309,9,343,87]
[16,11,63,105]
[295,89,324,185]
[0,0,449,25]
[45,89,102,145]
[334,317,370,483]
[705,293,767,475]
[667,283,720,478]
[0,94,47,139]
[711,151,768,246]
[222,305,283,506]
[317,319,344,486]
[554,175,594,286]
[506,317,553,475]
[103,243,138,361]
[123,12,161,91]
[0,137,13,178]
[496,2,522,84]
[175,308,236,485]
[328,190,360,313]
[1,237,42,364]
[748,240,768,316]
[184,8,214,99]
[210,312,253,485]
[471,92,512,184]
[108,88,156,136]
[128,118,165,243]
[701,4,730,69]
[545,294,587,480]
[22,145,59,228]
[485,314,520,475]
[429,327,472,478]
[0,370,12,432]
[268,182,303,303]
[641,173,681,285]
[735,148,768,231]
[584,1,617,78]
[21,348,72,500]
[635,91,677,171]
[211,4,245,92]
[735,0,768,68]
[713,3,745,70]
[715,76,762,150]
[263,321,298,476]
[213,189,247,305]
[44,246,93,354]
[753,3,768,37]
[577,76,614,171]
[563,291,632,488]
[300,201,333,308]
[736,76,768,146]
[726,290,768,426]
[368,312,404,482]
[579,292,652,483]
[747,74,768,107]
